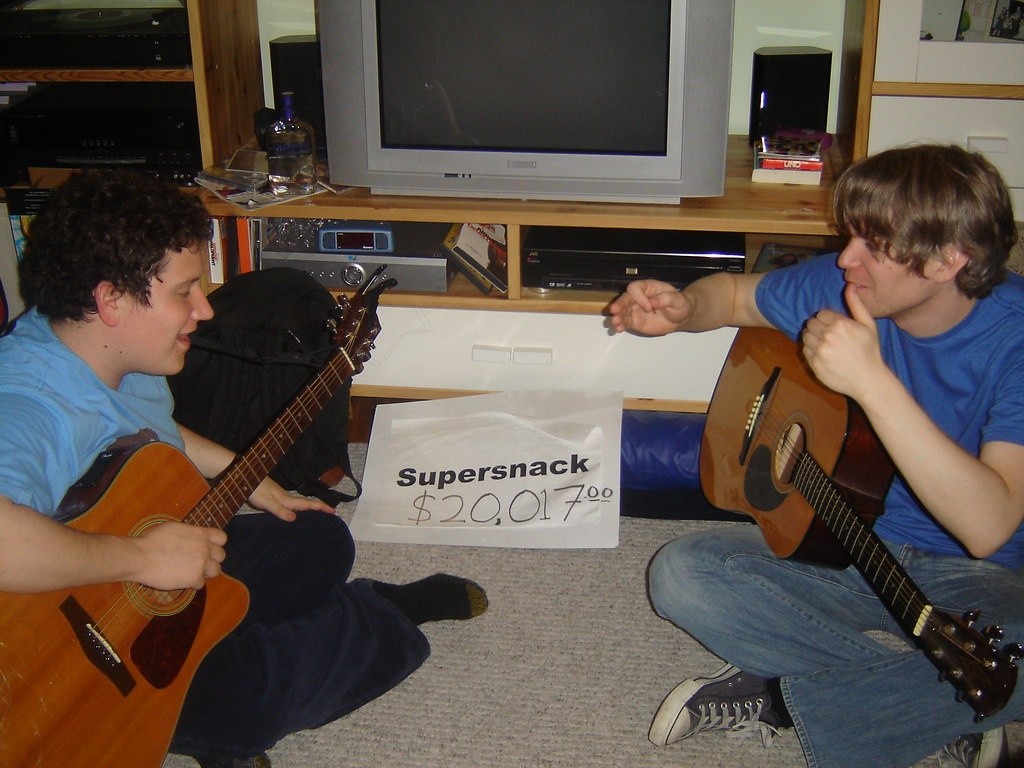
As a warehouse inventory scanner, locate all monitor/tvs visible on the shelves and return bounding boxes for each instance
[315,1,735,207]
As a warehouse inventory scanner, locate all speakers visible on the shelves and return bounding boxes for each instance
[270,34,325,148]
[746,46,833,145]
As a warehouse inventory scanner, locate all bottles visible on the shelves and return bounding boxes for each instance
[266,91,318,197]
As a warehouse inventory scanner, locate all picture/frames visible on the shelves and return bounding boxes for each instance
[956,0,1024,44]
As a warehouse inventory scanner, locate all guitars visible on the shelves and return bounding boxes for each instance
[698,326,1024,725]
[0,265,398,768]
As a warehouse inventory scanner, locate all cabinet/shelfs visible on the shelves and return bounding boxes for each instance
[1,0,1024,444]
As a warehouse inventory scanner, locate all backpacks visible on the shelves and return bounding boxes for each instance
[166,266,362,508]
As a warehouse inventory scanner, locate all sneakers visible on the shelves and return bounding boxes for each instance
[649,663,781,748]
[943,724,1009,768]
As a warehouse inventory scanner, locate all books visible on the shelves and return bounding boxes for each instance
[752,137,823,186]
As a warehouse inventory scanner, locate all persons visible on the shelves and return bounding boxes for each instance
[0,171,489,768]
[611,144,1024,768]
